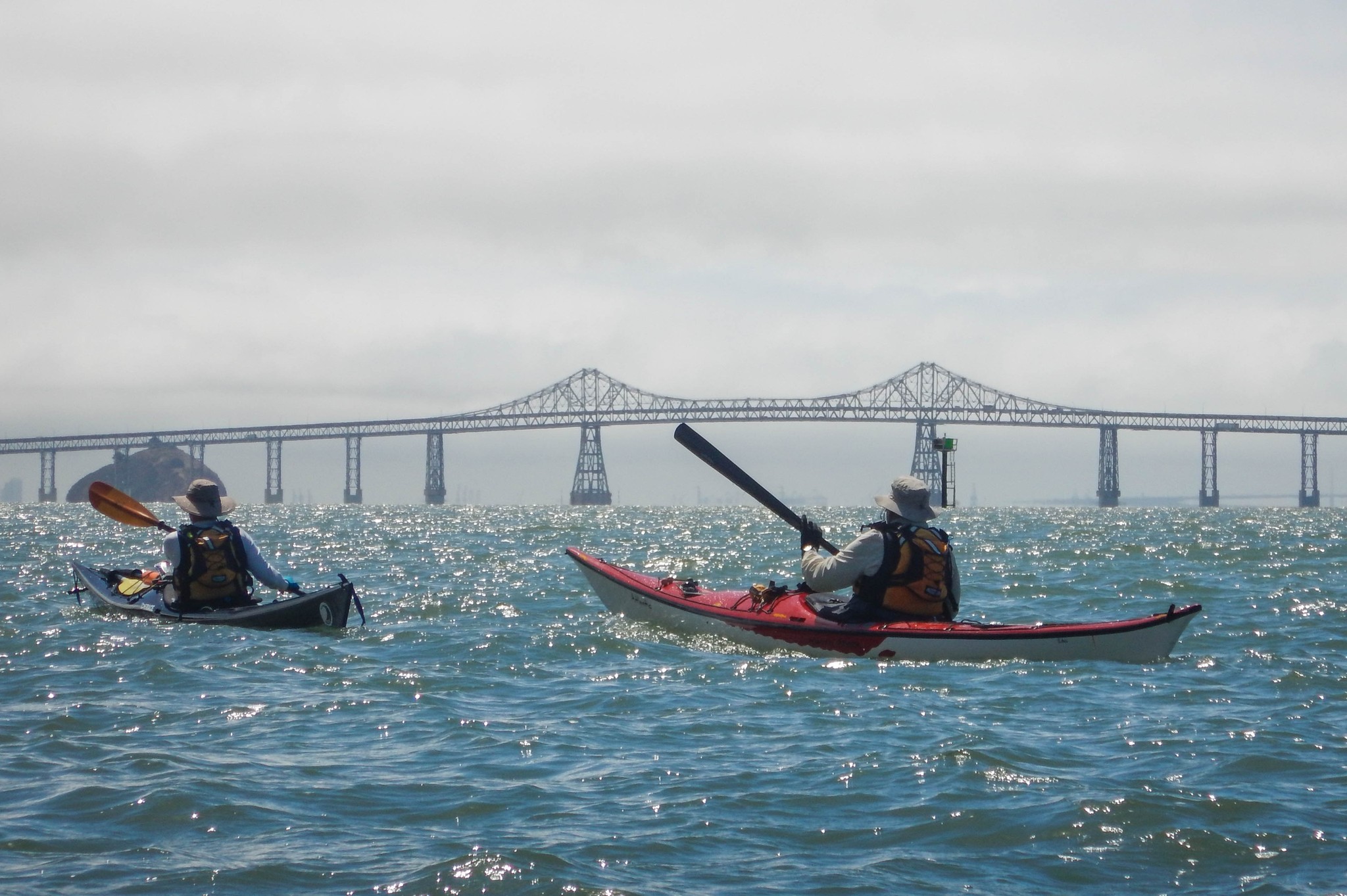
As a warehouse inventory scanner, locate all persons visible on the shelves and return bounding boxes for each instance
[799,476,961,623]
[162,479,300,613]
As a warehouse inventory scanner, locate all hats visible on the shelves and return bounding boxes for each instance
[874,475,941,522]
[171,479,236,517]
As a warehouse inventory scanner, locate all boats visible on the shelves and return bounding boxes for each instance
[563,544,1205,668]
[70,559,354,633]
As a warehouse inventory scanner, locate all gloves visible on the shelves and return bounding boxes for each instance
[800,513,823,554]
[283,579,301,593]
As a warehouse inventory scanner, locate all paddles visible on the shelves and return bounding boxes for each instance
[673,420,840,560]
[86,480,306,596]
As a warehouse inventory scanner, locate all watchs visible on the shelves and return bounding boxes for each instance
[802,543,819,556]
